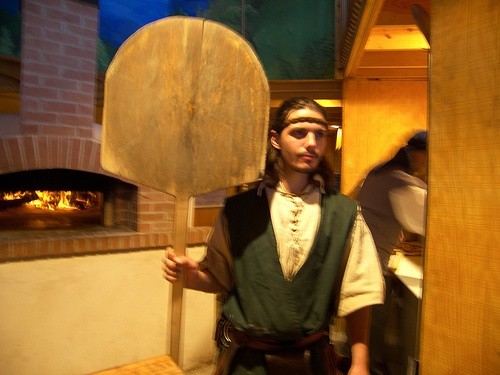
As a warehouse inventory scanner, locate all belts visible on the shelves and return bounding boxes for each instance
[225,325,327,356]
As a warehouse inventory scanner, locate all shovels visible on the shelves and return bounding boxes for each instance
[101,16,270,365]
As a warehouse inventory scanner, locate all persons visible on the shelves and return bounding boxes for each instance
[166,97,385,375]
[340,131,429,375]
